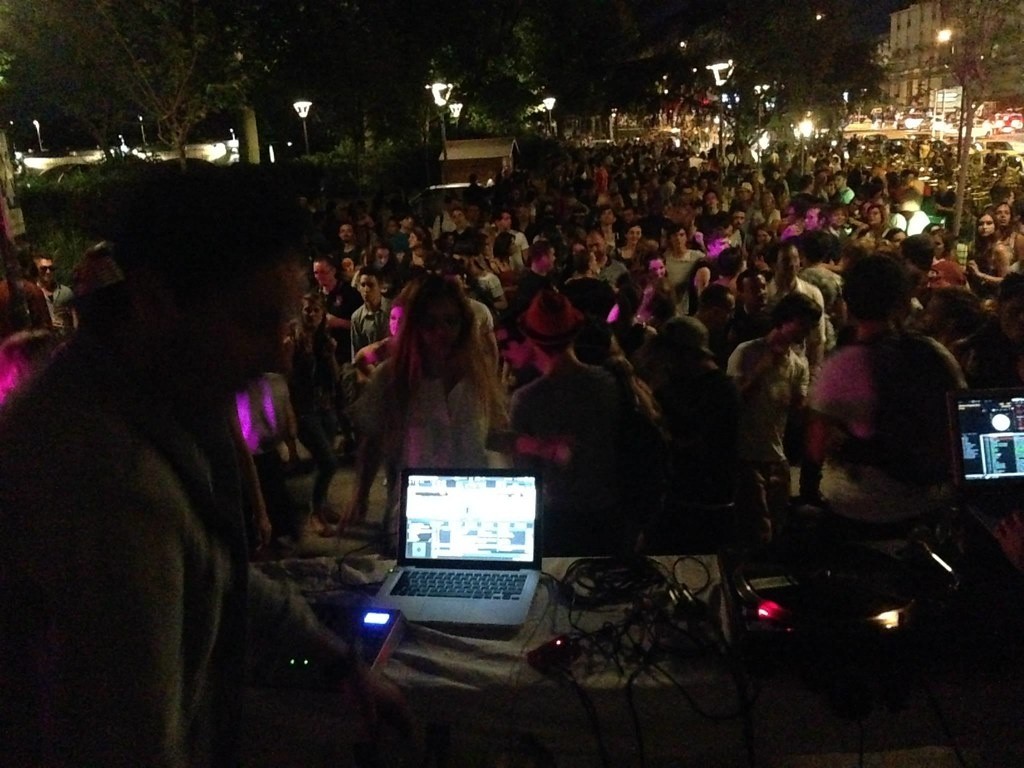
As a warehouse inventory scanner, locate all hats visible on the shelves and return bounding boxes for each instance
[515,292,585,347]
[658,316,715,357]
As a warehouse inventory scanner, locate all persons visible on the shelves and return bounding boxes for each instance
[0,157,425,768]
[1,133,1024,560]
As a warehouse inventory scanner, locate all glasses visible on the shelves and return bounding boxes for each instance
[452,258,469,265]
[39,266,56,273]
[311,267,334,276]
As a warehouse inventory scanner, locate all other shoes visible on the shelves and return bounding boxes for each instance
[310,500,341,523]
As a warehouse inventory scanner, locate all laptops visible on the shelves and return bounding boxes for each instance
[372,467,543,630]
[947,387,1024,529]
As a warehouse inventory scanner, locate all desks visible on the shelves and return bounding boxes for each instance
[235,554,1024,768]
